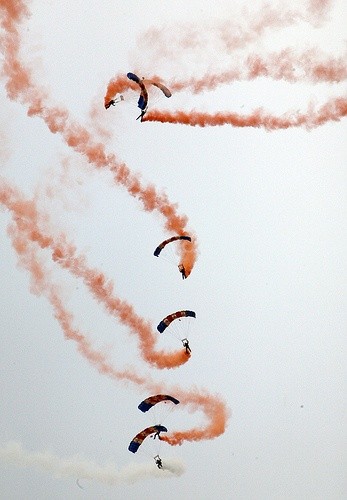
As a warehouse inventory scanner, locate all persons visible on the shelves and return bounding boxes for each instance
[182,340,191,353]
[179,266,187,280]
[105,100,115,109]
[156,458,163,469]
[136,110,146,122]
[154,432,160,439]
